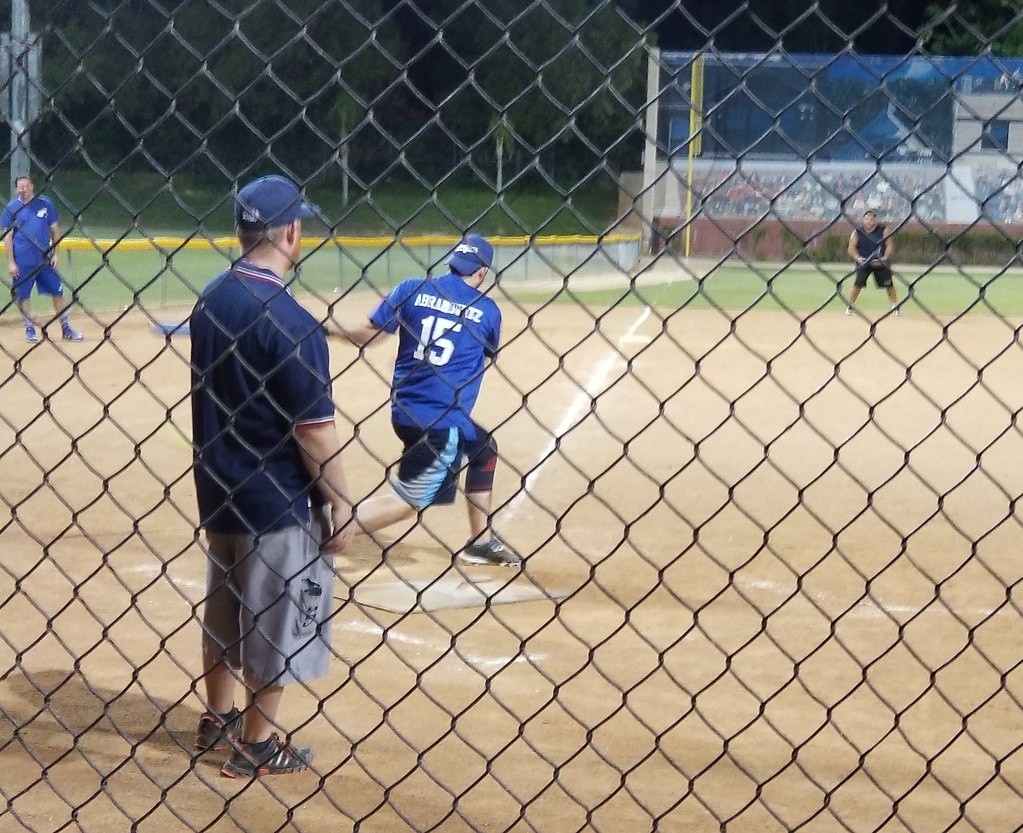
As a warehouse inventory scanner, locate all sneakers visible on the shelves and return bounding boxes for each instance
[220,731,315,778]
[25,325,38,343]
[458,532,522,567]
[193,701,245,750]
[61,325,84,342]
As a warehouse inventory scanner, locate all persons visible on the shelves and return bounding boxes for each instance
[846,212,902,317]
[188,177,357,777]
[0,176,85,342]
[322,235,523,568]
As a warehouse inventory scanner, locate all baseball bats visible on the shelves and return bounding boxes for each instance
[146,320,189,341]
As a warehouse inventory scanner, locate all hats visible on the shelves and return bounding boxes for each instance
[234,174,321,228]
[447,235,494,275]
